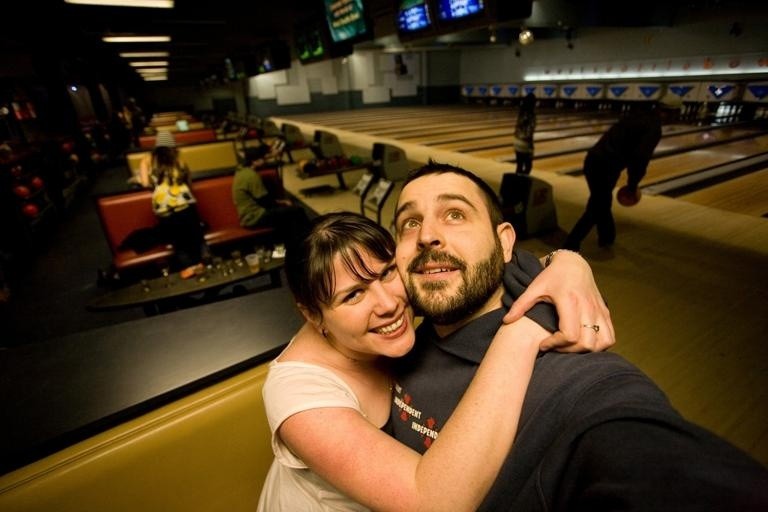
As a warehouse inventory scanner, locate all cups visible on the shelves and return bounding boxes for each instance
[245,254,259,274]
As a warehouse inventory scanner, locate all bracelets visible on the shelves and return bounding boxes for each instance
[544,248,580,266]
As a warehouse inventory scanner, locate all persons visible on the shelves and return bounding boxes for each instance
[513,93,538,175]
[563,101,670,261]
[390,161,768,512]
[233,148,310,247]
[255,213,617,512]
[126,146,204,280]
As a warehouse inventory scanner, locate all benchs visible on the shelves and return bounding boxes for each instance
[1,99,423,510]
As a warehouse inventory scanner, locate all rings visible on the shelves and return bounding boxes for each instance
[583,324,600,332]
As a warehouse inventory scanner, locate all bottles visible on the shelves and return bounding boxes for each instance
[256,232,284,264]
[195,259,245,282]
[139,279,151,293]
[162,268,170,289]
